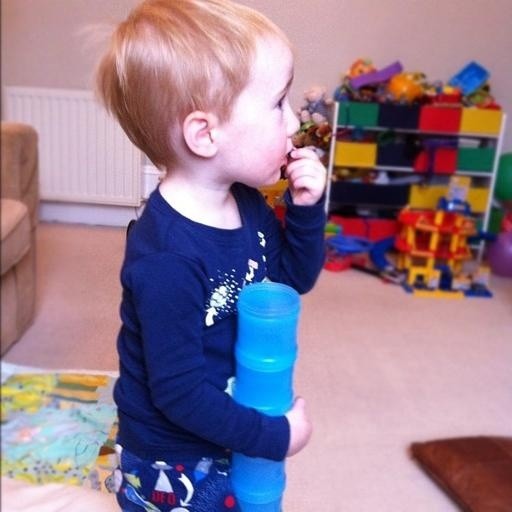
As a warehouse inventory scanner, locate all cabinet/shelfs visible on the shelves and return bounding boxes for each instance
[324,97,507,270]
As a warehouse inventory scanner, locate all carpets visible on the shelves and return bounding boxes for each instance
[0,362,124,512]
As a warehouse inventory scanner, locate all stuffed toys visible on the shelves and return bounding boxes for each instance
[253,86,332,213]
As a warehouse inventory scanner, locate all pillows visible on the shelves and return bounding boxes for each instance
[410,434,512,512]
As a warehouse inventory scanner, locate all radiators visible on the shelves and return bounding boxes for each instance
[4,85,146,208]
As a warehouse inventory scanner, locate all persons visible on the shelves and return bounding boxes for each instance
[86,1,326,512]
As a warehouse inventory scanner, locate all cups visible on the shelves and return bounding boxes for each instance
[231,280,302,512]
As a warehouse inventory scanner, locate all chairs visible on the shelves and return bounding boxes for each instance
[0,122,40,360]
